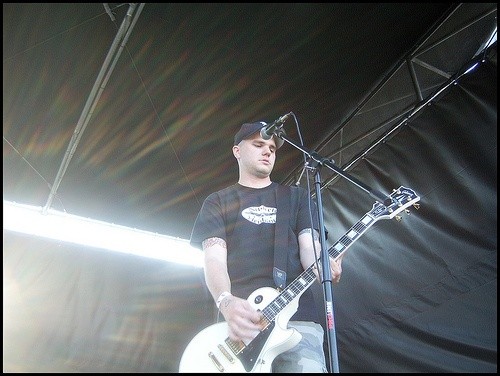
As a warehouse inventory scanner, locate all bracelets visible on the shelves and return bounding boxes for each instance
[216,292,234,312]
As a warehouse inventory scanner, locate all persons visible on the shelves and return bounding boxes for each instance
[188,121,341,372]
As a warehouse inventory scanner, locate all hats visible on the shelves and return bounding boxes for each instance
[233,122,285,150]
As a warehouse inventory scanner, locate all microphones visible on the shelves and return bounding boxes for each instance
[259,112,291,141]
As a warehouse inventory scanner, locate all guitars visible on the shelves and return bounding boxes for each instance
[178,186,422,374]
